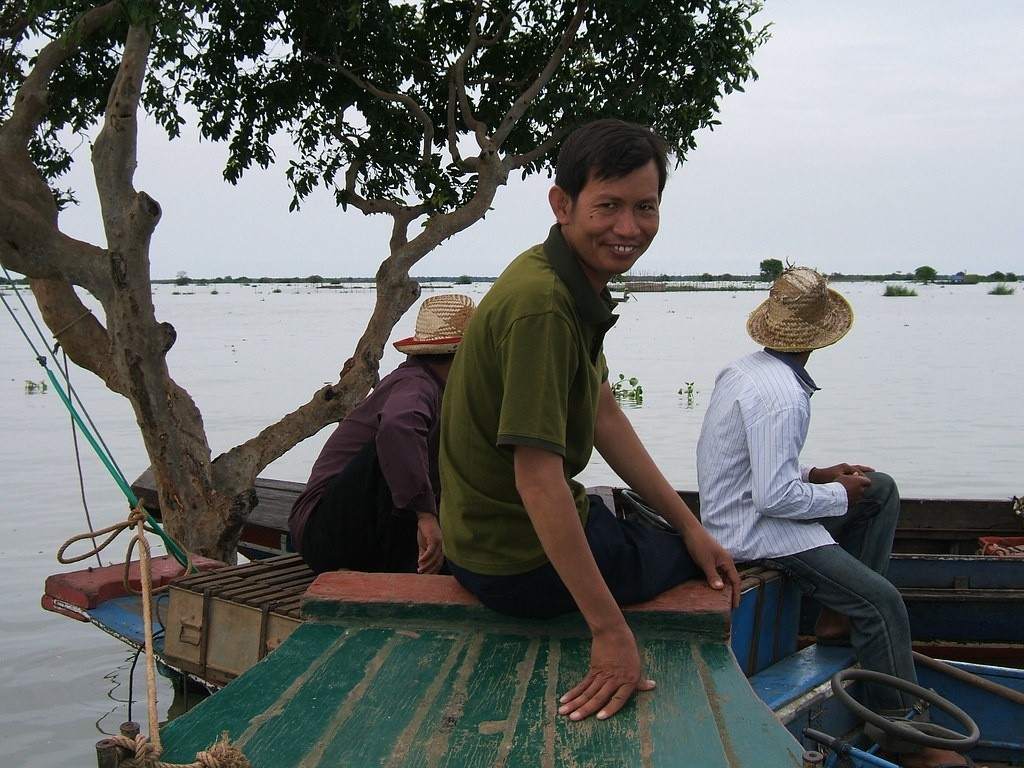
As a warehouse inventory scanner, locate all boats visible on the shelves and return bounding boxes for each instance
[45,445,1022,767]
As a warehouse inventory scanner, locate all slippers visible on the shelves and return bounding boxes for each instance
[936,754,976,768]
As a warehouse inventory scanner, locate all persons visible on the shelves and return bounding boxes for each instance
[696,268,986,768]
[285,295,450,575]
[439,118,740,721]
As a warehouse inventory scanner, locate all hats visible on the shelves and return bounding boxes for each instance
[747,267,855,353]
[392,294,478,354]
